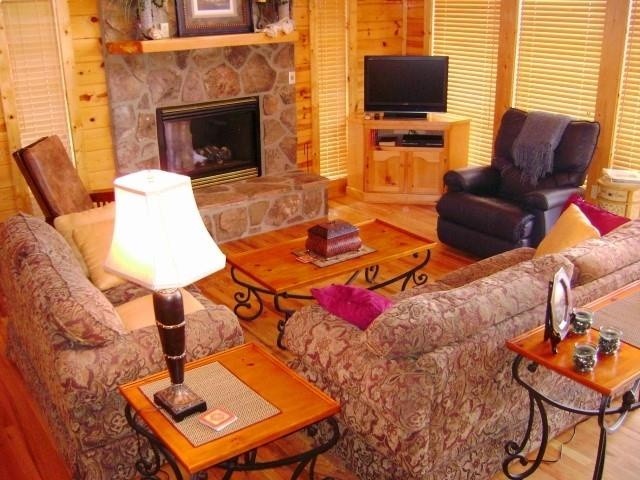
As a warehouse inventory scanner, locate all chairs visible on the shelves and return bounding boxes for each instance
[15,134,116,225]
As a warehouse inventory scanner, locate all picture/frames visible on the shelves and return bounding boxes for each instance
[174,0,256,37]
[542,265,575,356]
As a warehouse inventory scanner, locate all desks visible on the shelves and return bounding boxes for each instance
[117,340,343,479]
[597,174,640,220]
[505,280,639,480]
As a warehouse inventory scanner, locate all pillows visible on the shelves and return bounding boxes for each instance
[532,204,600,257]
[73,219,130,292]
[115,288,204,333]
[563,194,631,237]
[311,285,391,329]
[53,202,115,278]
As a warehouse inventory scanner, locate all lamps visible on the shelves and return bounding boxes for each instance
[103,169,226,423]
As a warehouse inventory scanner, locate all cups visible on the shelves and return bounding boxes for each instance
[570,340,599,374]
[598,324,623,356]
[571,308,594,335]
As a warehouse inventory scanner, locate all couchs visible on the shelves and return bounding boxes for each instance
[436,106,601,257]
[1,199,247,479]
[281,219,639,480]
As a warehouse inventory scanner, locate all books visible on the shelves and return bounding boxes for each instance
[601,168,640,184]
[378,137,396,147]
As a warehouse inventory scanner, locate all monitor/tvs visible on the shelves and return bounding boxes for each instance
[363,55,450,119]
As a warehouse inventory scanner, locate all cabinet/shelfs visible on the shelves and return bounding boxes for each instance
[346,111,470,202]
